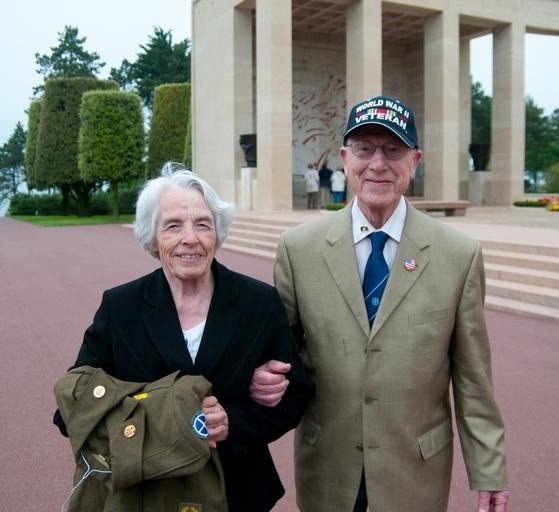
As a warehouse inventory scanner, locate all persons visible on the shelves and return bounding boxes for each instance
[248,97,510,512]
[304,164,320,209]
[319,164,333,207]
[53,160,315,512]
[329,167,346,203]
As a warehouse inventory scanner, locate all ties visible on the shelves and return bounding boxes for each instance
[361,231,390,331]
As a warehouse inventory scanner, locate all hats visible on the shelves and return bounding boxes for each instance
[341,95,419,150]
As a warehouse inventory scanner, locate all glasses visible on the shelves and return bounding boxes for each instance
[345,139,415,160]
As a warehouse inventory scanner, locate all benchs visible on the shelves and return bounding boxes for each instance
[408,201,471,217]
